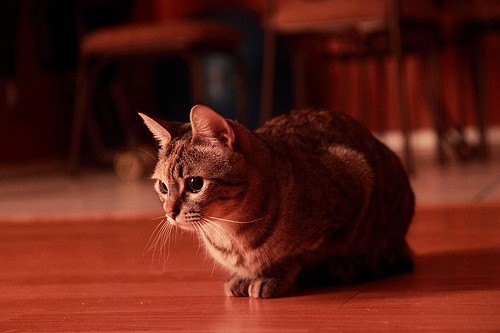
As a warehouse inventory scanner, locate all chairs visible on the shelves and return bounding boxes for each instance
[259,1,488,176]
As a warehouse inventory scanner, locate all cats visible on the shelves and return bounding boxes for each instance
[136,105,417,300]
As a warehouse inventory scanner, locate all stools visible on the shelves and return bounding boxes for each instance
[69,24,249,179]
